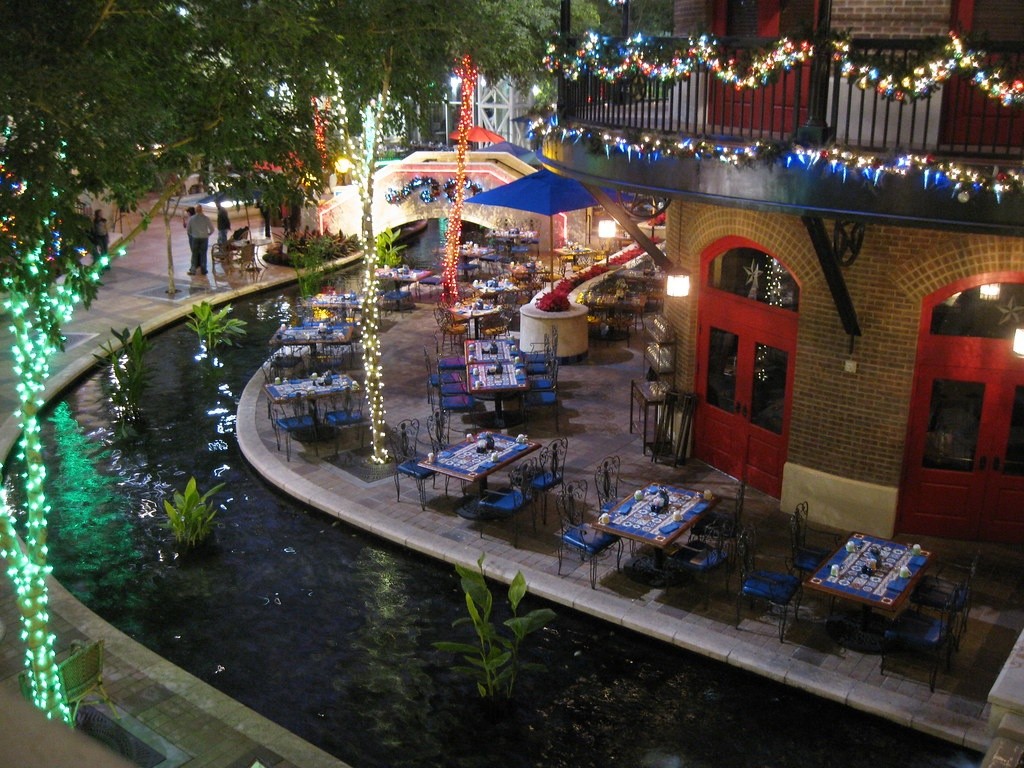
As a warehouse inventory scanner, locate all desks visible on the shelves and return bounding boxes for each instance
[506,265,551,303]
[262,374,362,441]
[469,284,520,305]
[552,248,597,273]
[485,230,538,256]
[374,268,433,311]
[590,482,722,589]
[803,531,937,655]
[270,325,354,379]
[417,429,543,521]
[231,238,272,270]
[448,303,502,339]
[464,339,531,429]
[616,270,665,312]
[433,245,494,282]
[587,293,647,341]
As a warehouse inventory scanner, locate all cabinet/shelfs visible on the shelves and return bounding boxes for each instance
[630,326,678,455]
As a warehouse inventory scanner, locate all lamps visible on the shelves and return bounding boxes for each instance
[598,209,616,237]
[667,196,690,297]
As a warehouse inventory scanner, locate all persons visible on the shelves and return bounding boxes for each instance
[94,209,109,256]
[260,199,270,237]
[214,199,231,252]
[183,204,215,275]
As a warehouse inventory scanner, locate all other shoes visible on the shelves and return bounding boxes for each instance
[202,270,208,275]
[188,271,195,275]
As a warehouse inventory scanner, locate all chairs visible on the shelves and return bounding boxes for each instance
[262,218,980,691]
[212,230,249,275]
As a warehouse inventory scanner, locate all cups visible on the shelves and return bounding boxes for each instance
[634,490,643,501]
[490,453,499,462]
[599,513,610,524]
[310,372,318,380]
[352,380,359,388]
[846,540,856,553]
[468,344,482,388]
[899,566,913,578]
[703,489,712,501]
[510,334,522,376]
[428,453,435,462]
[912,543,921,556]
[275,377,281,385]
[466,433,473,442]
[516,434,524,444]
[673,510,683,521]
[830,564,841,577]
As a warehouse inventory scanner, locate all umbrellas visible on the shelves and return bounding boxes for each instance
[450,124,505,144]
[460,167,640,215]
